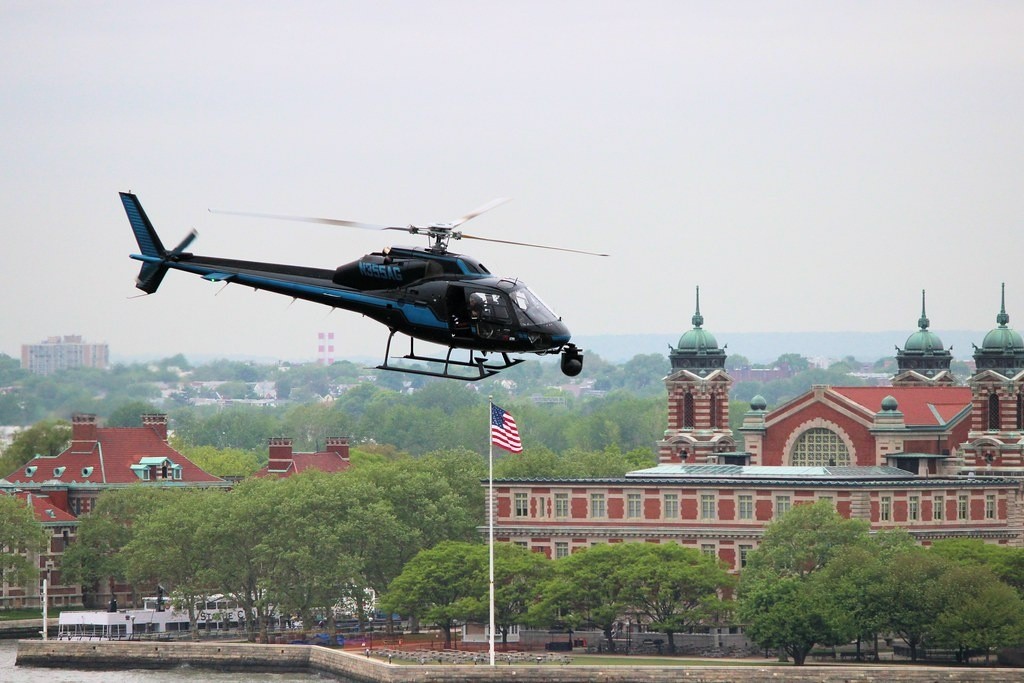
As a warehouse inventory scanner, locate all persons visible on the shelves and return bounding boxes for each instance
[471,294,485,319]
[498,296,518,317]
[492,403,523,454]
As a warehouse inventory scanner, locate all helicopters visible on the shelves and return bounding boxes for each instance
[123,188,611,382]
